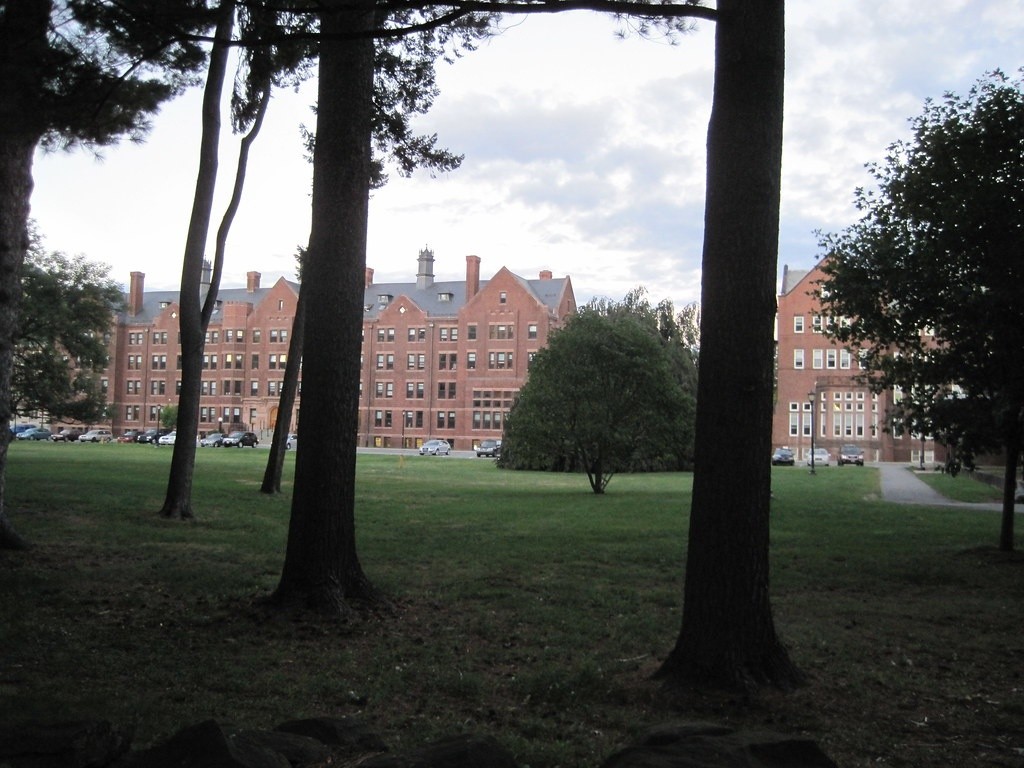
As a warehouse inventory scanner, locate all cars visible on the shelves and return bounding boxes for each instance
[50,429,86,443]
[476,438,502,458]
[158,430,177,445]
[78,429,114,444]
[199,432,228,448]
[117,430,143,443]
[270,434,298,450]
[419,439,452,456]
[807,447,832,466]
[136,429,170,444]
[15,427,54,442]
[771,446,796,466]
[10,424,38,435]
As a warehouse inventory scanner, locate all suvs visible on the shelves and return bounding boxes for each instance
[836,443,866,467]
[222,430,260,449]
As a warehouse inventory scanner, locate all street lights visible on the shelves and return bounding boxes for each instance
[156,404,162,444]
[807,389,817,475]
[401,409,406,449]
